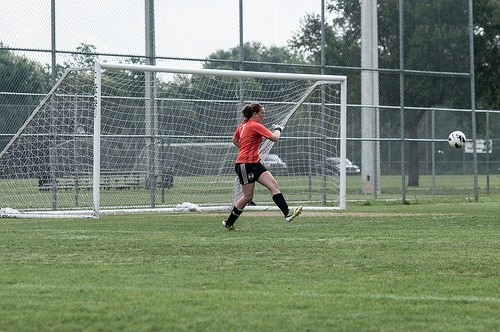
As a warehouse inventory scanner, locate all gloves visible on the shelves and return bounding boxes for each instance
[274,126,282,133]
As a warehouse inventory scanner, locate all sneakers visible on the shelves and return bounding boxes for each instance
[222,219,239,233]
[285,206,303,222]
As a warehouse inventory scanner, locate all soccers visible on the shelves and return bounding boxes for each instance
[448,131,466,149]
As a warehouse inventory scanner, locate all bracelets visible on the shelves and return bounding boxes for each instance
[274,127,282,132]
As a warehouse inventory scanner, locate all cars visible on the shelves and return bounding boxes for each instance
[260,154,288,176]
[326,157,360,176]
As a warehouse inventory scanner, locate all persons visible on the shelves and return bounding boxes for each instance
[222,104,302,233]
